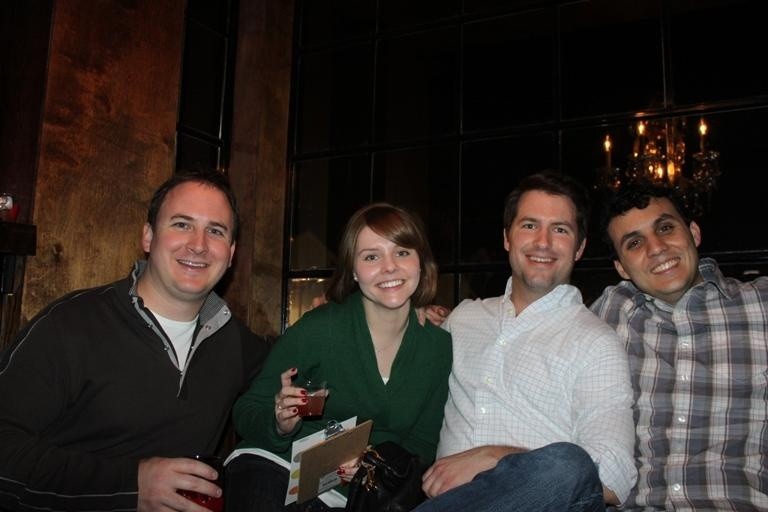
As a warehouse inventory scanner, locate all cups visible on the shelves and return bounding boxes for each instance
[175,452,225,512]
[293,377,328,416]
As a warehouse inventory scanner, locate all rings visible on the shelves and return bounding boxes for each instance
[275,398,285,410]
[277,389,290,400]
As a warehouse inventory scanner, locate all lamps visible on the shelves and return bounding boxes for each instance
[600,2,721,214]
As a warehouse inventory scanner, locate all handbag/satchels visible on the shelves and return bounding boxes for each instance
[344,439,429,510]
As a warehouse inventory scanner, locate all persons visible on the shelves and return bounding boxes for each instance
[427,174,767,511]
[230,202,453,512]
[306,170,639,511]
[0,162,427,512]
